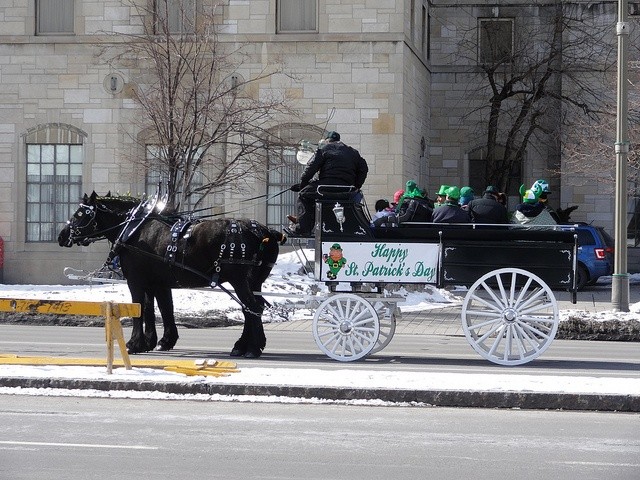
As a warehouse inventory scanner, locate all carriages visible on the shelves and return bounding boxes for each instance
[58,185,577,367]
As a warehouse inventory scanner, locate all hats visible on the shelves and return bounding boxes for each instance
[406,180,418,193]
[460,196,474,205]
[483,186,496,193]
[435,185,450,195]
[460,187,475,194]
[325,131,340,140]
[394,191,404,202]
[445,186,460,200]
[519,184,542,203]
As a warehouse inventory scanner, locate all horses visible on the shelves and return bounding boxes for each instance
[76,190,289,351]
[58,191,266,358]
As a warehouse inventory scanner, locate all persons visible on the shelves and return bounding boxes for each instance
[431,186,470,229]
[392,190,405,209]
[513,184,558,231]
[496,193,506,206]
[531,179,556,221]
[459,186,474,209]
[434,185,450,208]
[472,186,509,229]
[399,180,431,228]
[291,131,368,232]
[370,199,397,227]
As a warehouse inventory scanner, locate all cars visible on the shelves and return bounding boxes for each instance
[577,222,615,292]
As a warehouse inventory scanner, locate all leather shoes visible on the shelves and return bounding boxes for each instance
[287,215,296,224]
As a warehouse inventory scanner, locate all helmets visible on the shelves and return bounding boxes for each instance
[531,180,552,193]
